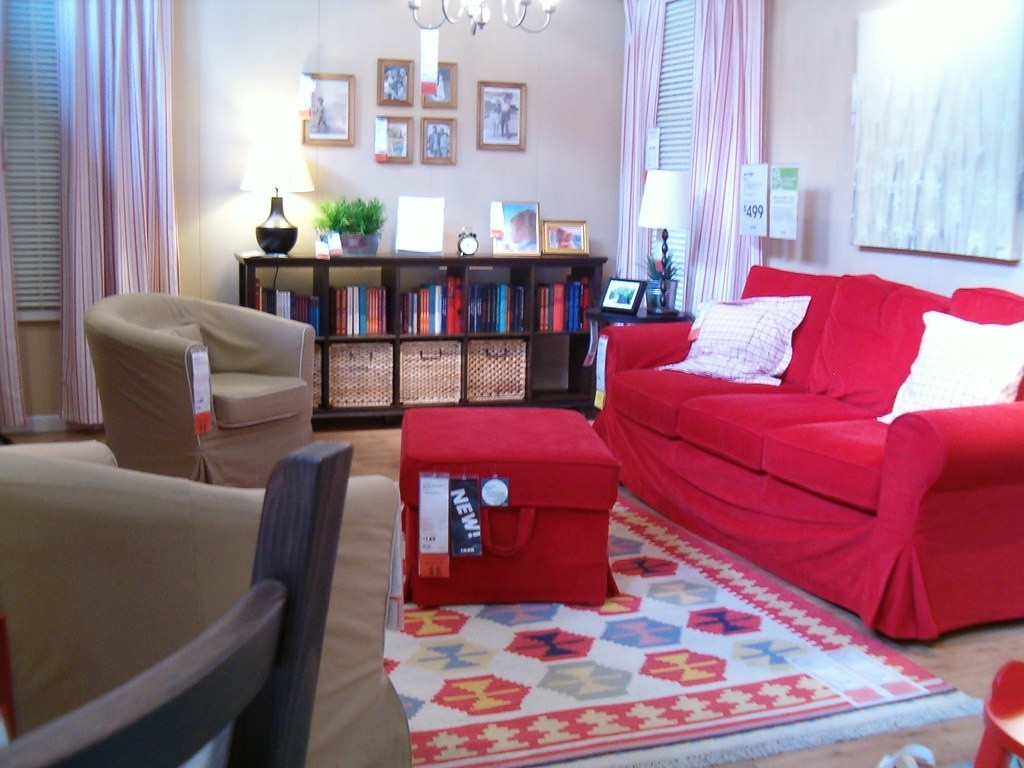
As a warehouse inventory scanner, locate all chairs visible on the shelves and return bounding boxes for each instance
[0,439,355,767]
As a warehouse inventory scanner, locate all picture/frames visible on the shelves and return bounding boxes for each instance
[421,61,458,108]
[420,117,456,164]
[598,277,647,316]
[376,58,415,106]
[300,72,356,148]
[376,115,413,163]
[542,220,590,255]
[492,201,542,256]
[475,80,527,152]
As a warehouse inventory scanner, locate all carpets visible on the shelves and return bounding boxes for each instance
[383,488,988,768]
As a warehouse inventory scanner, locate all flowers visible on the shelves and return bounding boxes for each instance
[646,252,683,280]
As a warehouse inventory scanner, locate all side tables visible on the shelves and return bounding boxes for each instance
[586,306,692,326]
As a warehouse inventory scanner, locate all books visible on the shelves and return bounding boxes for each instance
[536,279,592,331]
[326,284,387,335]
[255,285,320,337]
[466,281,524,331]
[399,276,465,334]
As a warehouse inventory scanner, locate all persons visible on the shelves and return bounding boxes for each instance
[428,125,451,157]
[385,66,409,101]
[501,210,538,252]
[314,97,329,130]
[491,97,511,139]
[552,228,572,248]
[435,73,451,100]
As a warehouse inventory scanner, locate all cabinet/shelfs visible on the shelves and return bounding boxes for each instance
[233,252,609,432]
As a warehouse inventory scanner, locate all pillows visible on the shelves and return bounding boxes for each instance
[877,311,1024,426]
[654,296,811,386]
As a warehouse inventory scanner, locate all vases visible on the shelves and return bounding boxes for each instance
[645,280,678,312]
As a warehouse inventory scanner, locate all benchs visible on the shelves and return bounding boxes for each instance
[398,407,621,610]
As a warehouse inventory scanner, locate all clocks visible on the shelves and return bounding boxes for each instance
[457,230,479,257]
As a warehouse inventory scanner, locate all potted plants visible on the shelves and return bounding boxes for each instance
[313,197,390,257]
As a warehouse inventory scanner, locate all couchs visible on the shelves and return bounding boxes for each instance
[83,291,316,488]
[0,439,414,767]
[592,265,1024,640]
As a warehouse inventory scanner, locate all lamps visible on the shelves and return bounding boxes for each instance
[409,0,556,36]
[637,170,691,316]
[239,142,315,254]
